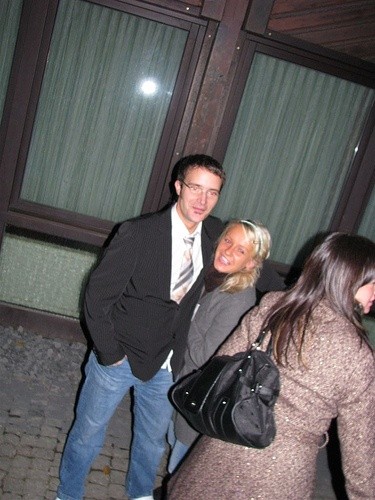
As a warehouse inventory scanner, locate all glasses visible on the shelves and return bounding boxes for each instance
[180,180,220,198]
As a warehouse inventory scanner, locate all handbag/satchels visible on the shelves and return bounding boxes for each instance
[167,348,282,450]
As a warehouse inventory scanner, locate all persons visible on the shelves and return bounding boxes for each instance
[167,214,270,476]
[167,232,375,500]
[54,155,291,500]
